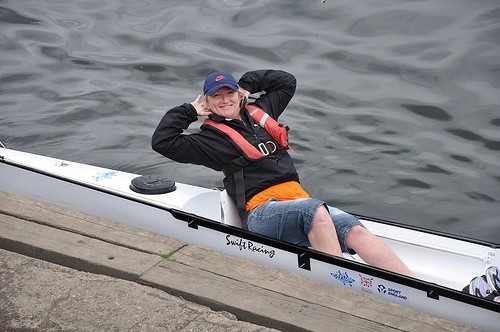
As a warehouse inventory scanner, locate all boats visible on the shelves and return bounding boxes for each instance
[0,140,500,332]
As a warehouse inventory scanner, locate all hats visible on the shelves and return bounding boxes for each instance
[204,72,238,96]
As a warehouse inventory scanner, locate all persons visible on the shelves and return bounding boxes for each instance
[150,69,413,278]
